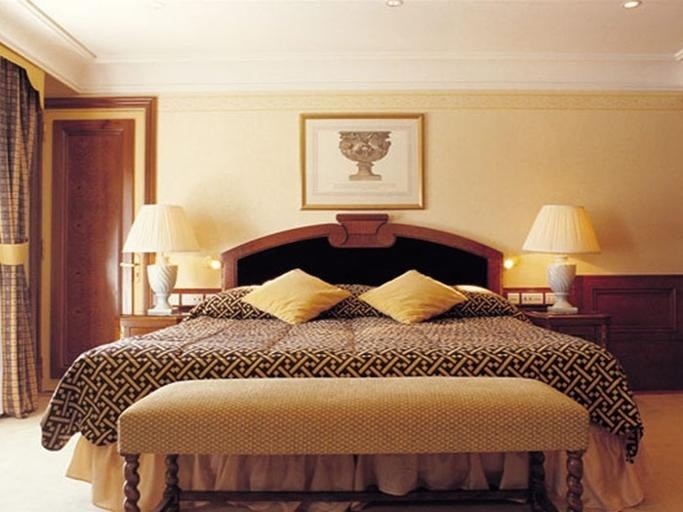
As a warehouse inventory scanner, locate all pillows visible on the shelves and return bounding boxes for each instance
[239,265,472,325]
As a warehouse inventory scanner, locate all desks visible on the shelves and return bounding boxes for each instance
[115,311,188,336]
[526,308,612,354]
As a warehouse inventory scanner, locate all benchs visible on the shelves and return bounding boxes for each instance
[106,372,591,512]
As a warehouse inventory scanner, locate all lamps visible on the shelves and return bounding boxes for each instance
[122,204,203,320]
[520,204,603,314]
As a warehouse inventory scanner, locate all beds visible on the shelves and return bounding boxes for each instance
[53,212,646,511]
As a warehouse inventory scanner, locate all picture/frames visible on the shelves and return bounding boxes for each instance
[295,110,427,214]
